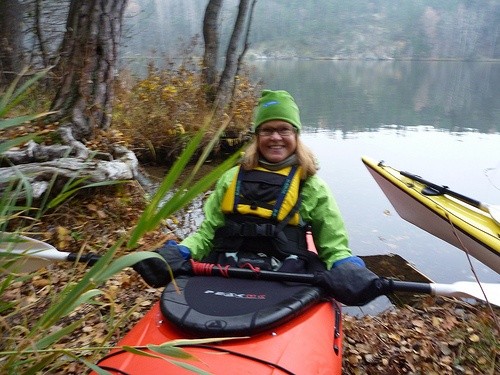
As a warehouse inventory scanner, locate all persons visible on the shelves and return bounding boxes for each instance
[152,89,367,282]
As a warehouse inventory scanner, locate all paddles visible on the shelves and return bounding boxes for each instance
[0,231,500,307]
[378,158,500,223]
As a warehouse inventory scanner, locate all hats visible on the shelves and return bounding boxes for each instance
[251,88,301,129]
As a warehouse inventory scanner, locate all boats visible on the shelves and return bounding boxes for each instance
[361,153,500,277]
[67,226,346,374]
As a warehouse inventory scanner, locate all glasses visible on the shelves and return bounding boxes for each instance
[252,124,298,137]
